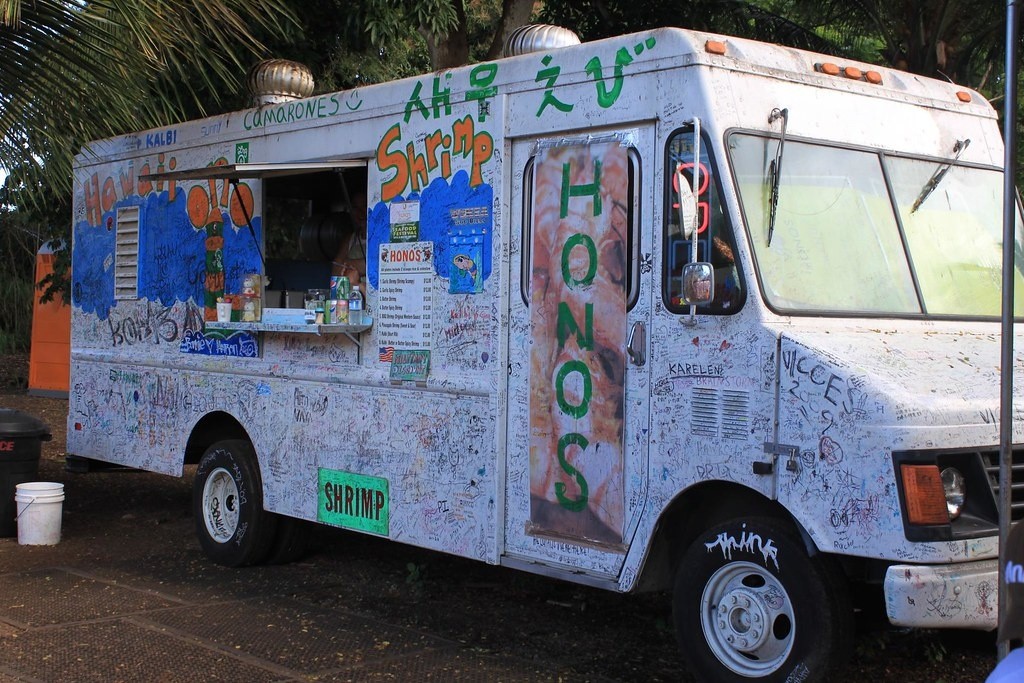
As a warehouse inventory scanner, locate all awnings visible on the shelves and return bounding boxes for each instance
[136,159,368,182]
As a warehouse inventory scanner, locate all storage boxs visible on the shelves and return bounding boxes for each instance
[262,307,309,324]
[282,290,304,308]
[265,291,281,307]
[239,274,261,322]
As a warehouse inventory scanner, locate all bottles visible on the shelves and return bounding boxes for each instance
[349,285,363,325]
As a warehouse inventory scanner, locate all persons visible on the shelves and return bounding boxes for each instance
[331,203,366,299]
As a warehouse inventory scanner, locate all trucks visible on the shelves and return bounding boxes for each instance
[65,27,1023,682]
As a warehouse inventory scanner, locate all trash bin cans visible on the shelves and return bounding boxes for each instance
[0,407,55,538]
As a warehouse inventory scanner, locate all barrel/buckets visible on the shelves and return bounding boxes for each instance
[15,481,65,547]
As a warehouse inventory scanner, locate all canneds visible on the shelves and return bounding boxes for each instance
[325,276,349,324]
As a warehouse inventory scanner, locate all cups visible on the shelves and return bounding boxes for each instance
[216,303,232,322]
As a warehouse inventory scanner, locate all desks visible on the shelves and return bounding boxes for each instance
[205,314,373,365]
